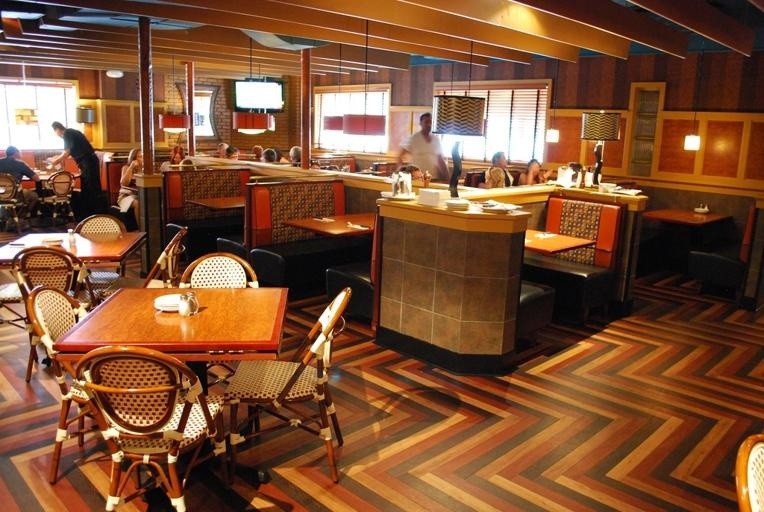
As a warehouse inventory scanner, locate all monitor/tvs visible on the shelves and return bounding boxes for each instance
[232,79,285,114]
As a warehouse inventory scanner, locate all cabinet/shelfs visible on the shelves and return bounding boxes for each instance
[373,194,534,375]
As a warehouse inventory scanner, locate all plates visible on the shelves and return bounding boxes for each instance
[380,192,415,201]
[446,199,470,210]
[154,294,180,312]
[482,204,522,214]
[42,240,63,246]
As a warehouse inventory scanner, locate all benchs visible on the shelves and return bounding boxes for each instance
[209,152,764,350]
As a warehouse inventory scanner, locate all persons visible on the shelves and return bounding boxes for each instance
[51,122,105,219]
[0,146,40,230]
[226,146,239,160]
[478,152,517,189]
[398,165,406,173]
[160,146,185,173]
[519,159,553,185]
[404,165,421,180]
[252,145,301,166]
[397,113,447,183]
[218,143,229,159]
[117,148,142,229]
[179,159,196,171]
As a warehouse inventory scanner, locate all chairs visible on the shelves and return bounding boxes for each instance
[76,345,227,512]
[73,214,128,286]
[178,252,258,290]
[225,286,352,484]
[10,246,99,384]
[137,225,187,292]
[26,285,119,485]
[1,156,76,236]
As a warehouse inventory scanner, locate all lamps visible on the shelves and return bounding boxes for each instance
[431,94,486,198]
[158,56,190,135]
[683,36,705,152]
[545,59,561,144]
[579,110,622,190]
[311,20,386,159]
[231,36,273,135]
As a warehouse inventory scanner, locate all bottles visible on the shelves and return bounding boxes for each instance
[178,292,197,315]
[68,229,75,244]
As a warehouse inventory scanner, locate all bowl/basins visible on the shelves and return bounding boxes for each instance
[599,183,616,192]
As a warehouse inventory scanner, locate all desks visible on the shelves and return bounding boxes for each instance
[2,232,147,294]
[49,286,289,487]
